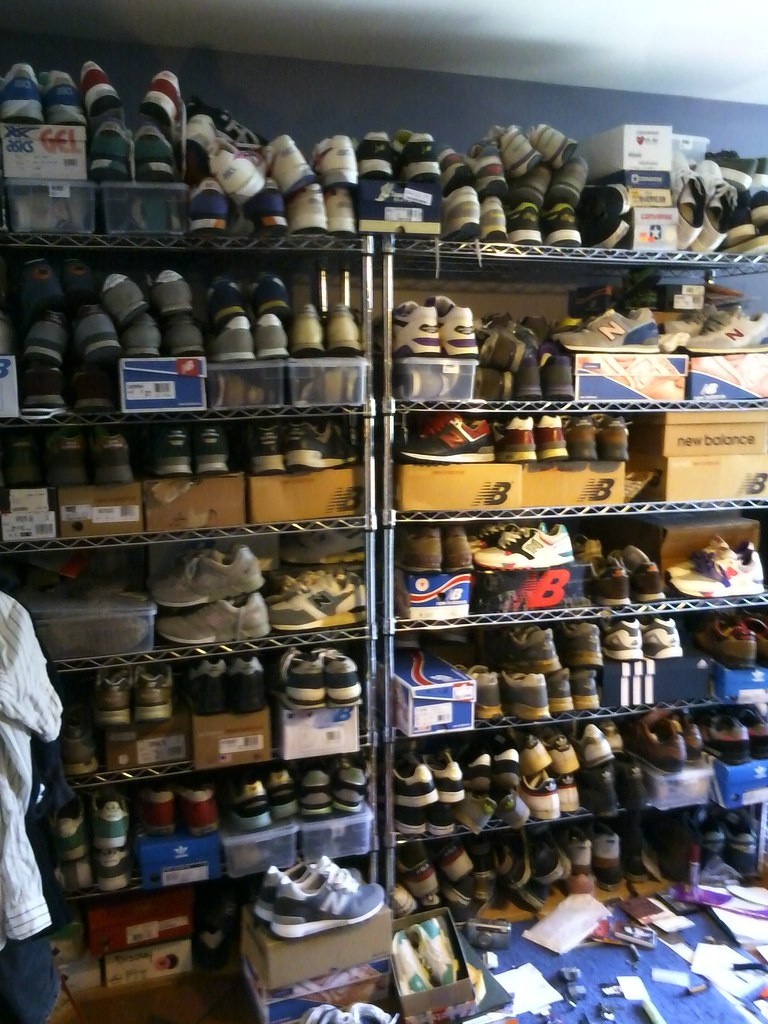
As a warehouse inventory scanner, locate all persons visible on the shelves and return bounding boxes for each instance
[0,589,63,1023]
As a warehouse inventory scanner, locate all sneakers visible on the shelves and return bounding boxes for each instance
[0,60,768,1024]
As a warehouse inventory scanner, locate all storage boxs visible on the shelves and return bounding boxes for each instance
[1,125,768,1022]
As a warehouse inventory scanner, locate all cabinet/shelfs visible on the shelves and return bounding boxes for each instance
[387,212,768,905]
[1,206,384,912]
